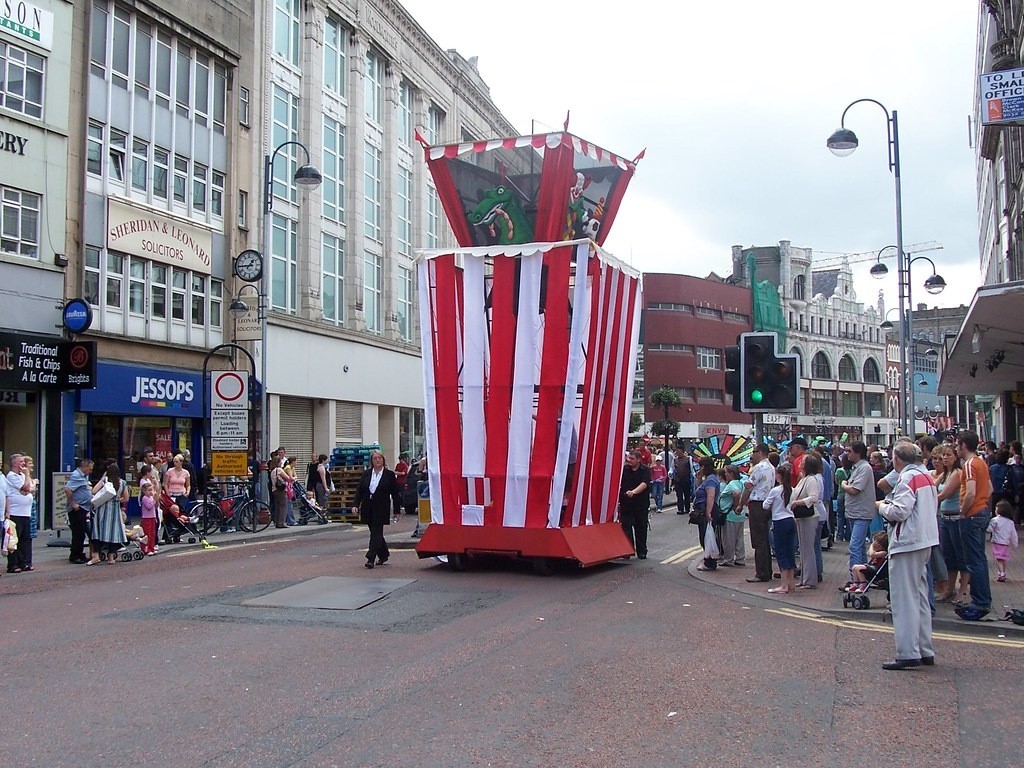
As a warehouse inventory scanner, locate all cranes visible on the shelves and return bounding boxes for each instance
[722,241,944,278]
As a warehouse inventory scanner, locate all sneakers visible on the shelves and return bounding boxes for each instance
[855,585,869,592]
[845,584,859,591]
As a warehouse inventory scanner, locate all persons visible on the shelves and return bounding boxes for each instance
[352,449,402,568]
[618,429,1023,671]
[0,453,39,572]
[392,452,427,523]
[65,446,218,565]
[876,443,941,669]
[240,447,336,526]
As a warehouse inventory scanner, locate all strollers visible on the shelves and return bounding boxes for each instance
[159,494,206,544]
[842,520,891,610]
[90,510,148,562]
[290,479,329,525]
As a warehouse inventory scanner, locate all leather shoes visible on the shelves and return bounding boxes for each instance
[922,656,935,665]
[882,658,919,669]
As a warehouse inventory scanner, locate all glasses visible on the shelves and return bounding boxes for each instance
[752,451,759,453]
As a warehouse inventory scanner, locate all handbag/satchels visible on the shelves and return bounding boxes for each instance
[687,510,707,525]
[704,520,720,559]
[1002,465,1014,493]
[793,504,814,518]
[2,517,18,557]
[90,474,116,509]
[275,480,286,490]
[712,512,726,526]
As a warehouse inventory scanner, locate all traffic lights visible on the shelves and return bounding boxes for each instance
[724,335,741,412]
[740,331,800,413]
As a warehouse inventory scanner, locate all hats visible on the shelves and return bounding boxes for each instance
[655,454,662,461]
[912,443,923,457]
[786,437,807,446]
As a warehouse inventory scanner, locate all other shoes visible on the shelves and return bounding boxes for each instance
[656,509,689,514]
[147,550,158,555]
[937,590,957,601]
[365,561,374,568]
[747,575,817,593]
[952,591,968,603]
[637,554,647,558]
[697,562,746,571]
[69,553,115,565]
[375,557,388,565]
[7,563,35,573]
[997,575,1007,582]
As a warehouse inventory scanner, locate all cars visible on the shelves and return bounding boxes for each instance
[402,464,428,514]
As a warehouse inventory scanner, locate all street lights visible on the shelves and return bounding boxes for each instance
[827,98,907,437]
[870,245,947,442]
[813,414,836,437]
[914,401,942,433]
[230,141,322,524]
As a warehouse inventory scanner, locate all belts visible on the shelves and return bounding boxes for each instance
[941,513,960,522]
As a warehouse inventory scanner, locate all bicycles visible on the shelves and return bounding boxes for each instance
[189,484,272,535]
